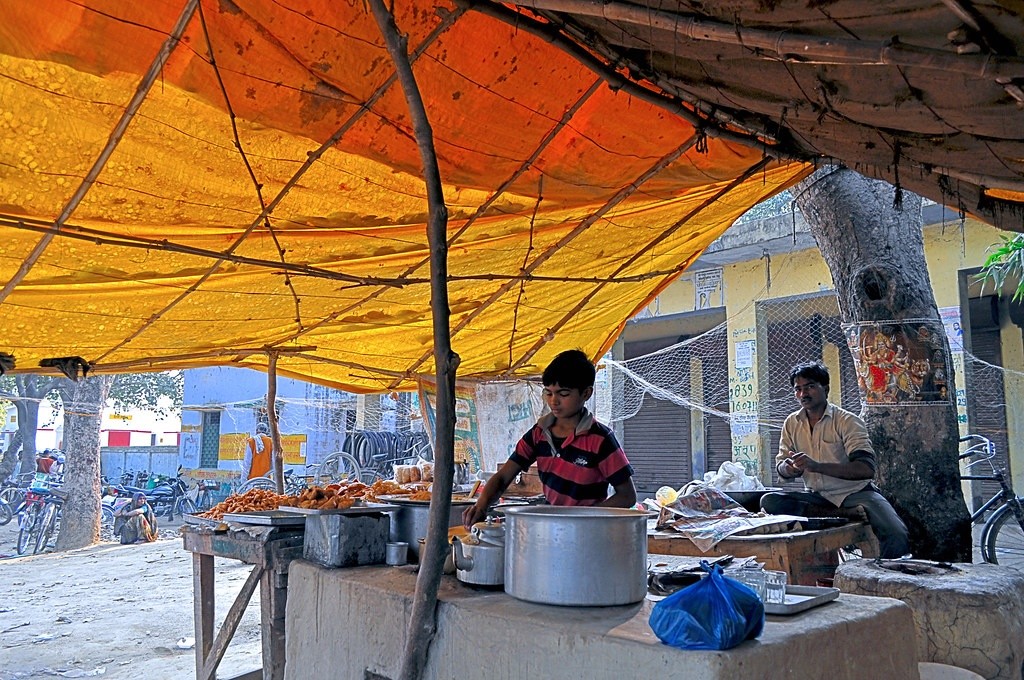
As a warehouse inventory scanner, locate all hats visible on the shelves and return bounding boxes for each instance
[57,456,66,463]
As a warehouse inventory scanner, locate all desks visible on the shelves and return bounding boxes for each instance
[182,525,302,680]
[284,551,921,680]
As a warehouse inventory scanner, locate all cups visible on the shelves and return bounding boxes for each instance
[701,569,787,605]
[386,541,409,565]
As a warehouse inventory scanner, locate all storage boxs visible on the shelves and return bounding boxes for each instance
[301,512,391,568]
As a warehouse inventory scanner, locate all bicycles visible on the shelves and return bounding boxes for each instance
[0,464,218,556]
[238,442,439,502]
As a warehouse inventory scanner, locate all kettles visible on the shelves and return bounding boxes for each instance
[450,516,506,585]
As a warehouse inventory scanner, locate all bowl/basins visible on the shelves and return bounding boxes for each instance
[386,502,470,554]
[494,505,655,607]
[416,538,455,575]
[721,488,784,513]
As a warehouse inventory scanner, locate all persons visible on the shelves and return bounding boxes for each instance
[113,493,158,544]
[462,350,637,531]
[11,451,23,480]
[761,362,910,558]
[36,449,65,482]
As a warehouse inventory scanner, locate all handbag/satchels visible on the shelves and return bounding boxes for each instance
[648,559,766,651]
